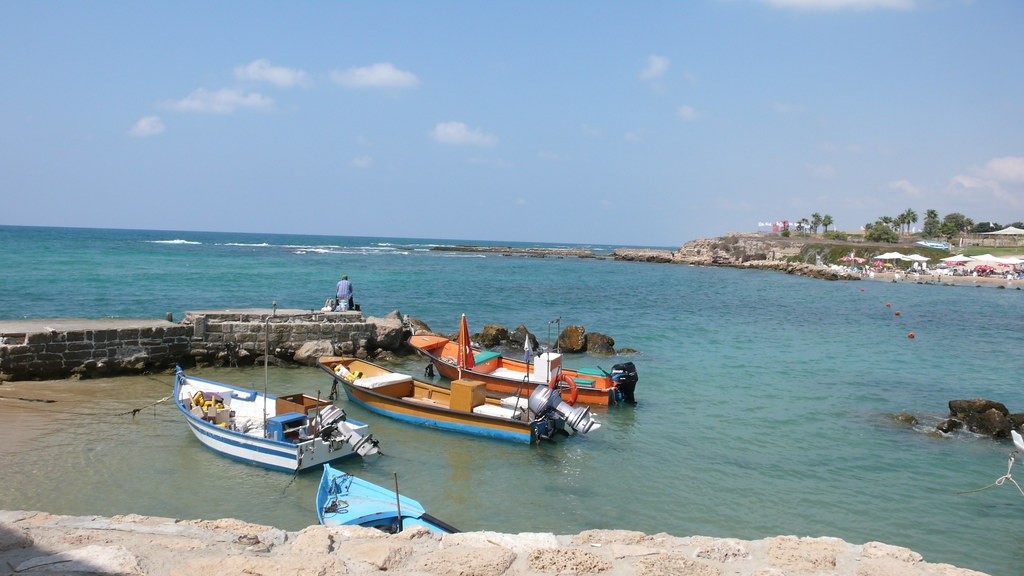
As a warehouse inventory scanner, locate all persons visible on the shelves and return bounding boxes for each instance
[931,274,941,283]
[335,274,354,311]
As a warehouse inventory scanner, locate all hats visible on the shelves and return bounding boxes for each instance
[342,275,347,279]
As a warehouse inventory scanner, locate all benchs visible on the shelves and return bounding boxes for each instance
[452,350,502,374]
[576,367,611,389]
[353,372,413,397]
[560,376,596,389]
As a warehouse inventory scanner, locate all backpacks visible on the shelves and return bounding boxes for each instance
[325,298,336,311]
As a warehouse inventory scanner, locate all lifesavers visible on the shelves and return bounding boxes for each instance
[548,373,578,406]
[203,400,212,411]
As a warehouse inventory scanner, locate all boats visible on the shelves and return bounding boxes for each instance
[315,461,459,535]
[407,317,639,407]
[172,300,383,476]
[315,353,596,447]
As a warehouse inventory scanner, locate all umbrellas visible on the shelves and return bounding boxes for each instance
[455,313,476,370]
[873,252,1024,271]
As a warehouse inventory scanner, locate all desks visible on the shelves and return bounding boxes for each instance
[449,378,486,412]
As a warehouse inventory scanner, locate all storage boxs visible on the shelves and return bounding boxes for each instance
[500,396,528,421]
[276,392,334,434]
[191,403,231,424]
[473,404,521,421]
